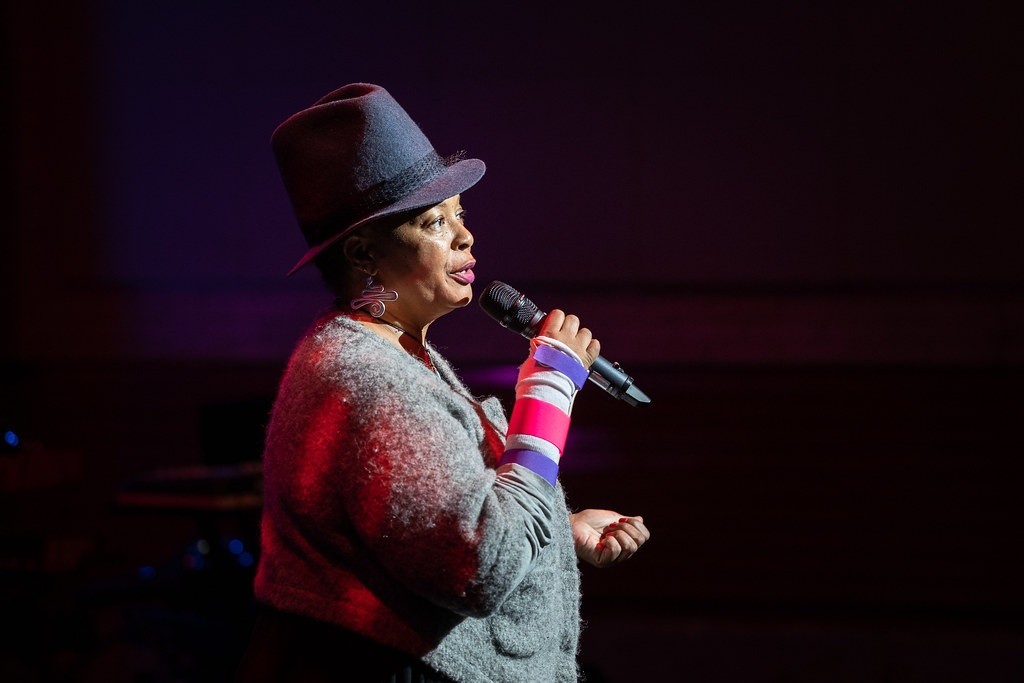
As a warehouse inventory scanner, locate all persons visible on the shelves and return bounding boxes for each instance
[252,82,650,683]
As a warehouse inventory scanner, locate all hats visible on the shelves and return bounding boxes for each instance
[269,82,486,276]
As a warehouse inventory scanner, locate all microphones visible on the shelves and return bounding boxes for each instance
[476,278,651,408]
